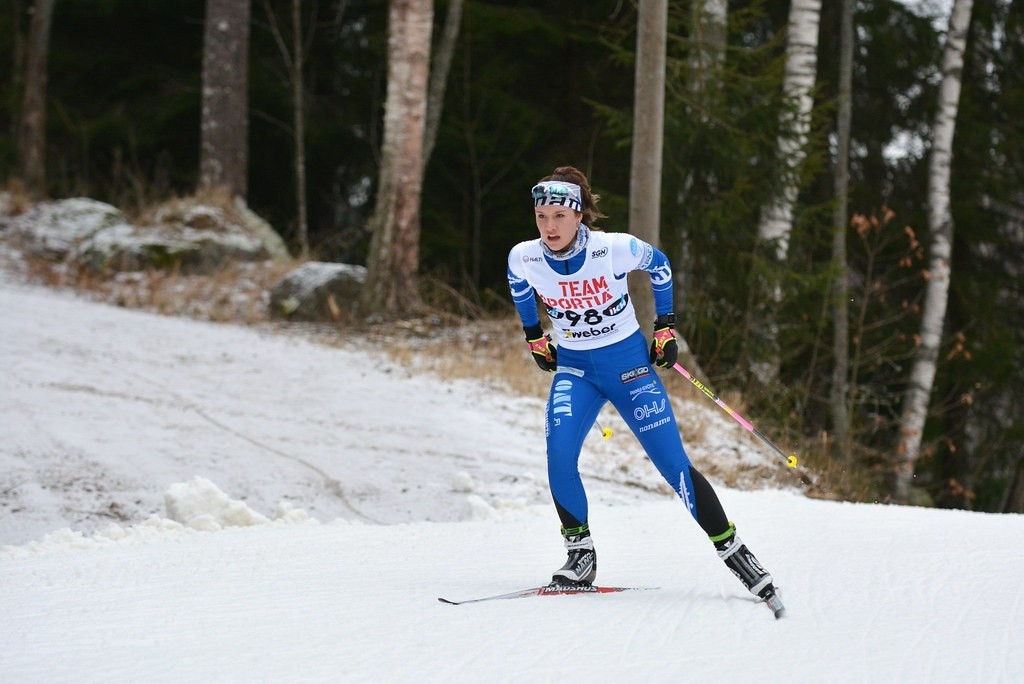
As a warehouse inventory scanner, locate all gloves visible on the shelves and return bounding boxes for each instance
[649,314,678,369]
[522,320,557,372]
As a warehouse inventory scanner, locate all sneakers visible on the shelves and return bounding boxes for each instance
[552,535,597,586]
[716,536,773,597]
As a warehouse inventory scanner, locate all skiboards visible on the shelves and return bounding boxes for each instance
[437,583,785,621]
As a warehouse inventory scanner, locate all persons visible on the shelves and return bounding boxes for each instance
[508,166,785,619]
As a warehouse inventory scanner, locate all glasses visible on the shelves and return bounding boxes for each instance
[532,184,580,204]
